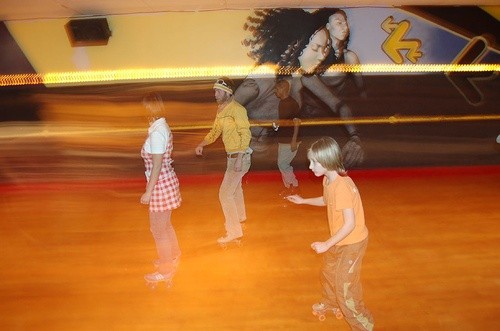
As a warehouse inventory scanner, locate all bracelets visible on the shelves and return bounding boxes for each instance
[351,133,360,137]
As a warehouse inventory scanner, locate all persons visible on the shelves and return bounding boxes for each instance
[196,77,254,250]
[287,135,374,331]
[272,79,302,199]
[139,92,182,284]
[232,7,367,170]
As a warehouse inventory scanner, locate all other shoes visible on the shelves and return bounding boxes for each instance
[282,186,302,199]
[278,188,289,196]
[217,236,244,243]
[223,218,246,226]
[144,271,172,281]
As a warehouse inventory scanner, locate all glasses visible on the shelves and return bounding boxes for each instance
[215,79,226,86]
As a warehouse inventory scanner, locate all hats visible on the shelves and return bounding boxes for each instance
[213,76,232,94]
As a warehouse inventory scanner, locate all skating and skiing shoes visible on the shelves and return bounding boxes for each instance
[312,303,343,322]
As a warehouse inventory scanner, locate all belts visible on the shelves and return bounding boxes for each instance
[227,153,249,158]
